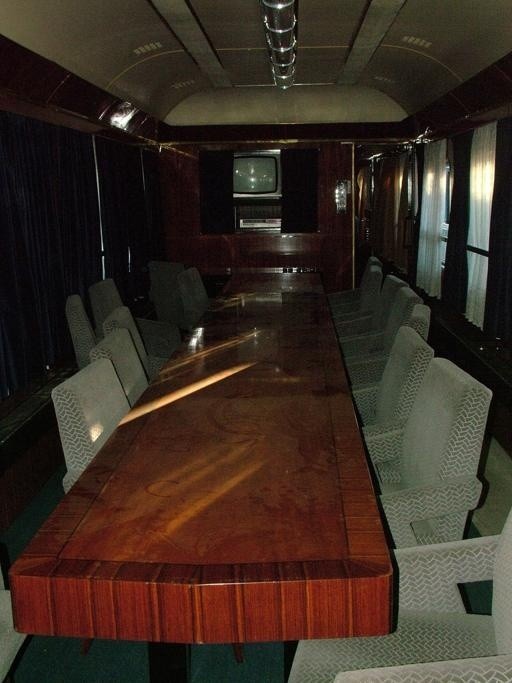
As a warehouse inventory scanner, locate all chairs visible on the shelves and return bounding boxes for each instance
[271,257,511,682]
[47,256,213,498]
[0,538,38,682]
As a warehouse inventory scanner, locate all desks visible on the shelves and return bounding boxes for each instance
[0,266,402,683]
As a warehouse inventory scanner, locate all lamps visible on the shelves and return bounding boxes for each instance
[260,0,299,91]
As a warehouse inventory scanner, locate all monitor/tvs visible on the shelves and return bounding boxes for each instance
[234,149,282,200]
